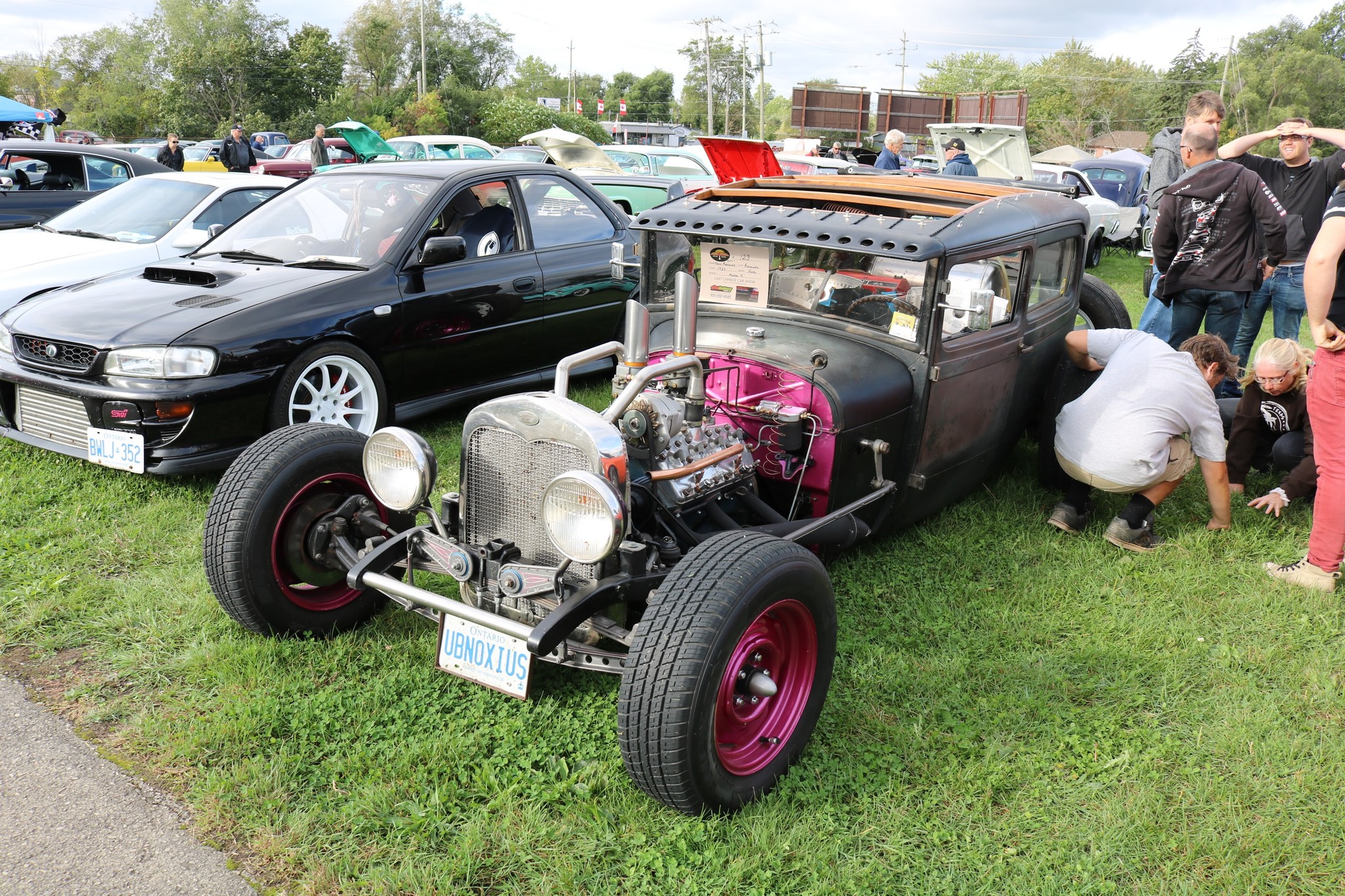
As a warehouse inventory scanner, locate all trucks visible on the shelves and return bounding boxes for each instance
[6,130,103,144]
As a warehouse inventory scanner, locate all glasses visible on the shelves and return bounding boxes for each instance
[170,140,179,144]
[1278,135,1308,142]
[237,130,243,132]
[835,148,840,150]
[1254,369,1290,384]
[1175,145,1193,152]
[70,140,72,141]
[945,148,956,152]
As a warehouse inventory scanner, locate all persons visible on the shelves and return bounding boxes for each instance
[805,144,822,157]
[1048,88,1345,593]
[941,138,978,177]
[311,124,330,171]
[252,135,264,152]
[823,141,848,162]
[66,135,95,145]
[522,139,533,146]
[157,133,185,172]
[873,129,905,170]
[219,124,257,173]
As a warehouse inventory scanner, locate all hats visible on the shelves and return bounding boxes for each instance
[81,136,88,139]
[941,138,966,151]
[813,144,822,150]
[231,124,245,131]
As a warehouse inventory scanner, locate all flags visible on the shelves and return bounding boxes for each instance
[612,115,617,133]
[598,99,604,115]
[577,100,583,115]
[620,99,628,117]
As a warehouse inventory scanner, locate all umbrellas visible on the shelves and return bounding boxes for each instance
[0,96,53,122]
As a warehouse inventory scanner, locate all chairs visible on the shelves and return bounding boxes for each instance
[453,203,515,258]
[906,287,1009,338]
[768,269,864,314]
[40,172,74,190]
[0,169,31,190]
[349,197,419,263]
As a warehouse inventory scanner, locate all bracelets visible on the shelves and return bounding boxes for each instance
[1269,487,1290,507]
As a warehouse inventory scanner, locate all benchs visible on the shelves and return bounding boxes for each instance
[71,177,120,191]
[870,255,1000,295]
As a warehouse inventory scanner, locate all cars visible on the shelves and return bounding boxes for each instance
[85,117,527,209]
[0,142,178,230]
[0,159,695,478]
[204,175,1091,822]
[0,173,404,293]
[489,124,1155,299]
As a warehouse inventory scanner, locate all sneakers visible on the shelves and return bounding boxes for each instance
[1046,500,1089,535]
[1102,512,1166,552]
[1262,552,1342,593]
